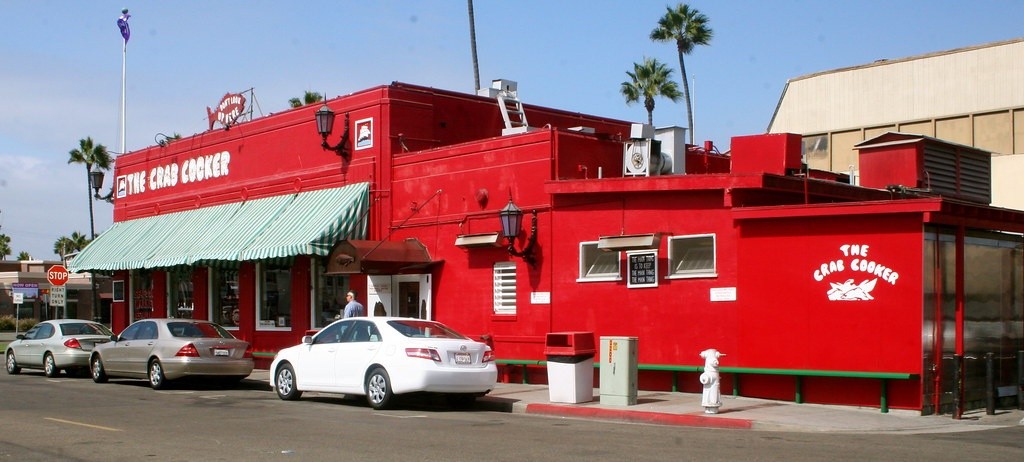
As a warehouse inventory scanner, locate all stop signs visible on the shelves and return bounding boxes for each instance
[47,264,69,287]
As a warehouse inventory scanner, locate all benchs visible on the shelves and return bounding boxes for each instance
[495,359,920,414]
[253,351,277,358]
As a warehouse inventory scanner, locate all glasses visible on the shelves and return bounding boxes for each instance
[346,295,351,297]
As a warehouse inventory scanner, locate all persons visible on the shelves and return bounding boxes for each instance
[343,289,363,319]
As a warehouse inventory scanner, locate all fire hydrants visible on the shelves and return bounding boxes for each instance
[697,348,727,414]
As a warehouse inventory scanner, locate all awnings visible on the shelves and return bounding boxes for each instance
[66,182,369,274]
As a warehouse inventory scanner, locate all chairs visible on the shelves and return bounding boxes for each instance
[355,328,372,341]
[79,325,92,335]
[182,326,200,336]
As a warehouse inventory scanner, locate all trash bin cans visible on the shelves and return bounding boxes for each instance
[543,331,597,404]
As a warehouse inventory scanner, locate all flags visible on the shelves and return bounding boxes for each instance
[117,13,132,51]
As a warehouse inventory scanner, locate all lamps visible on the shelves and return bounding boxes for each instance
[499,186,537,267]
[90,164,115,204]
[315,92,350,163]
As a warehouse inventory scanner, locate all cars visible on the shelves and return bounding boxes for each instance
[87,317,255,390]
[269,315,498,410]
[5,318,117,379]
